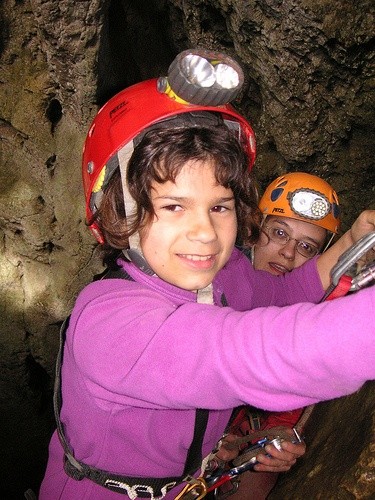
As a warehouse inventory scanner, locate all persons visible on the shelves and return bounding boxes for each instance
[39,49,375,500]
[221,172,340,500]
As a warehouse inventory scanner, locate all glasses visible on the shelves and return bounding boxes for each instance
[264,224,319,258]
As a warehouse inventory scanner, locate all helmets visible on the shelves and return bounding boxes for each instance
[258,172,340,234]
[82,78,256,245]
